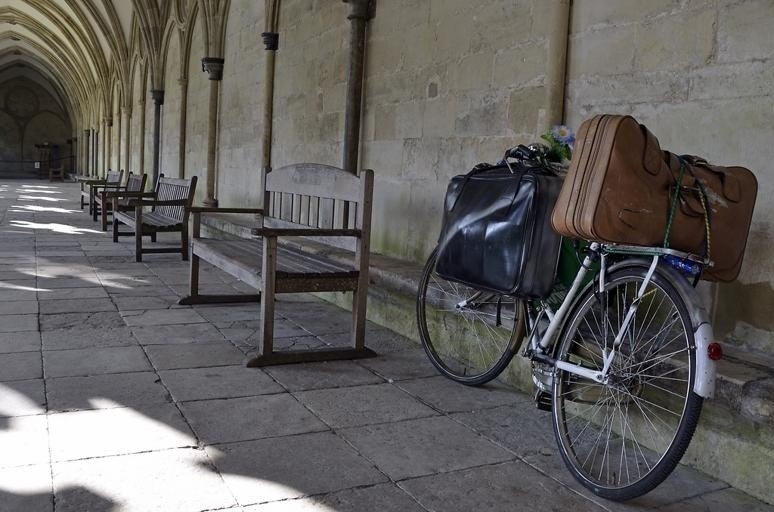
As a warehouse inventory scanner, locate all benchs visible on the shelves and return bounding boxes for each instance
[176,161,378,369]
[92,171,147,231]
[48,163,66,183]
[76,168,123,214]
[106,173,198,263]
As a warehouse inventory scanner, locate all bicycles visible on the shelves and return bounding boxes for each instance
[416,144,722,502]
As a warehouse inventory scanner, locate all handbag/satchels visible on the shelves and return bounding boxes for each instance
[435,150,563,299]
[551,115,758,283]
